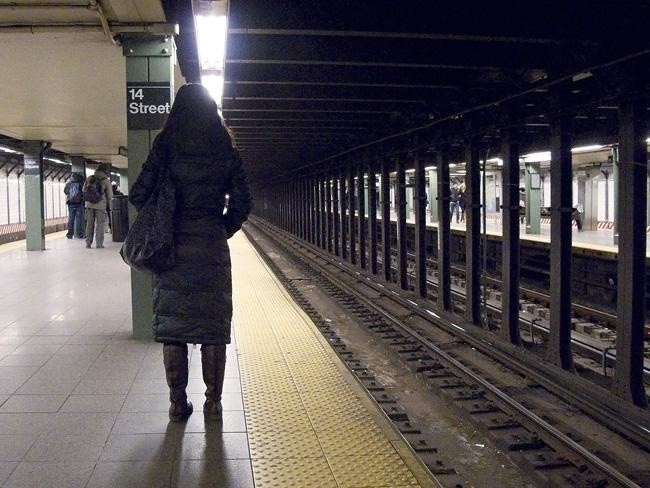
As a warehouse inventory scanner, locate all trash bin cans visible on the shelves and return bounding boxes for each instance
[110,195,129,242]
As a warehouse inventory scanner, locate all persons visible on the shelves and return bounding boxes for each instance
[450,182,461,223]
[80,162,113,248]
[459,183,467,222]
[107,184,124,231]
[63,171,86,239]
[127,82,255,422]
[519,187,526,223]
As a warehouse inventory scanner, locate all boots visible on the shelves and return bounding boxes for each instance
[201,344,226,421]
[163,342,193,422]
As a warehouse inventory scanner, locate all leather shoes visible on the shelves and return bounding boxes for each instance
[97,246,104,248]
[86,244,90,248]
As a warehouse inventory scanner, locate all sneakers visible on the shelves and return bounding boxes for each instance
[66,233,72,239]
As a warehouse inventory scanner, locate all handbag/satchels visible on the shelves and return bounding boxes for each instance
[120,132,177,273]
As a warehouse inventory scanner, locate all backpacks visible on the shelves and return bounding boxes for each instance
[82,175,106,204]
[68,180,83,204]
